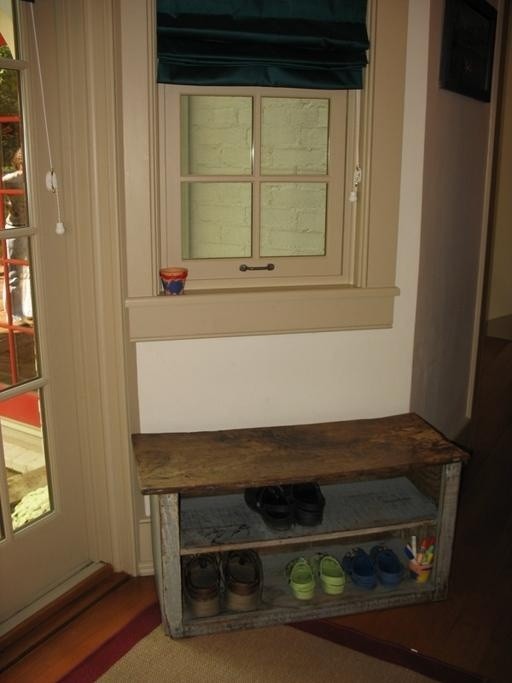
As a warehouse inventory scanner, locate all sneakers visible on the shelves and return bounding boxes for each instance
[222,550,264,611]
[181,553,221,618]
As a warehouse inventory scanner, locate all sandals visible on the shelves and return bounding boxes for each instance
[371,544,403,584]
[343,548,378,588]
[244,485,294,531]
[286,556,316,600]
[281,483,325,527]
[312,552,346,594]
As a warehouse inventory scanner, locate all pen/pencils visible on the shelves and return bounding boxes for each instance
[403,535,435,566]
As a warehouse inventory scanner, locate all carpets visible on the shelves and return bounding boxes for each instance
[57,603,485,682]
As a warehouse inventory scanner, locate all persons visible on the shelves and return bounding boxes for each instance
[0,144,33,323]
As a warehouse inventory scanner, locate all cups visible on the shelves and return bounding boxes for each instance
[161,267,188,295]
[410,560,432,584]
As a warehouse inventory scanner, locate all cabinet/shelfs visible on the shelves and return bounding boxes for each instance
[130,411,471,641]
